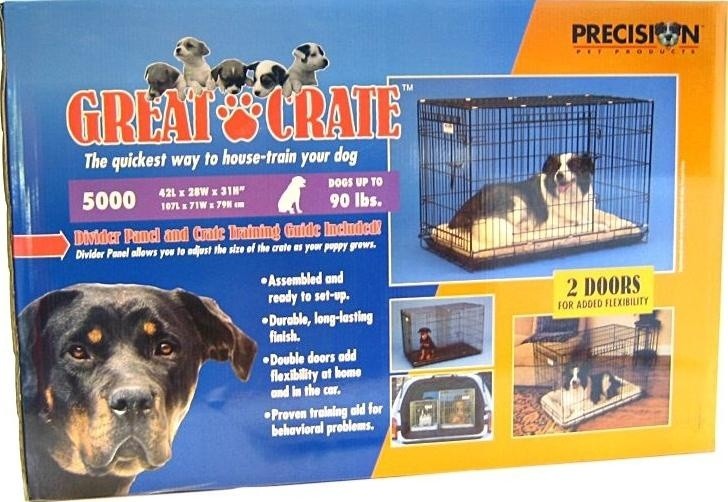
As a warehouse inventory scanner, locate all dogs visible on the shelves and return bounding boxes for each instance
[145,62,187,102]
[248,59,289,99]
[16,281,259,502]
[655,22,681,48]
[172,37,215,97]
[417,327,438,361]
[211,58,255,96]
[558,359,627,418]
[285,41,329,96]
[431,150,638,257]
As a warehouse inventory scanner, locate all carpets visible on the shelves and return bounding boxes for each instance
[513,386,577,437]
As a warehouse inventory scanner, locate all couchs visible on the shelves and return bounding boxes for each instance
[513,317,588,386]
